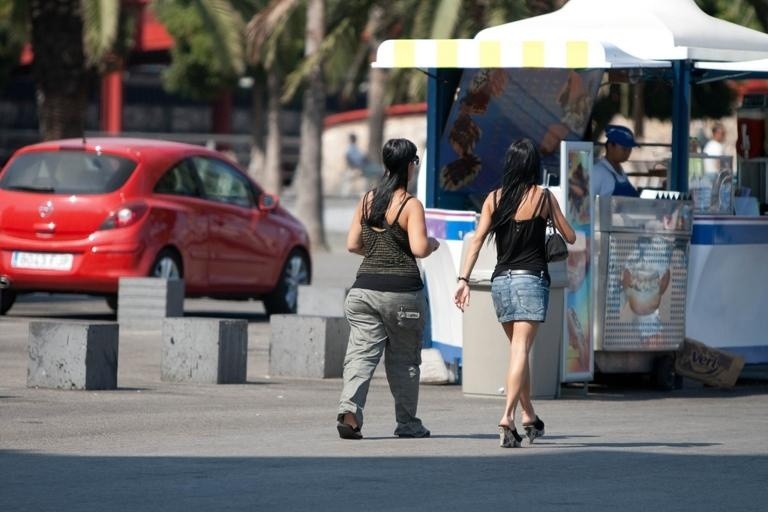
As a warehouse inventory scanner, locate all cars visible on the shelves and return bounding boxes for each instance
[0,136,312,315]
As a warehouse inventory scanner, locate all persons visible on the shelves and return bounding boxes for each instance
[454,138,576,448]
[592,124,641,200]
[345,134,387,182]
[333,138,439,439]
[702,123,726,184]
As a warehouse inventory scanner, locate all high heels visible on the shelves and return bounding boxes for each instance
[497,423,523,448]
[523,415,545,444]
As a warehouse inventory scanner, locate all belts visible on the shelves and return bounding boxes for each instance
[496,268,547,279]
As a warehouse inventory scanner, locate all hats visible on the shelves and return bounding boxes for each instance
[604,123,641,149]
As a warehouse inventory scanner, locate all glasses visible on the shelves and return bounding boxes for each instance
[412,155,420,166]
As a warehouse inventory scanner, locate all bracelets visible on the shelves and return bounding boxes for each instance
[457,277,469,283]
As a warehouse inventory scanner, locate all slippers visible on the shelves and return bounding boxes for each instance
[398,429,432,438]
[337,422,363,439]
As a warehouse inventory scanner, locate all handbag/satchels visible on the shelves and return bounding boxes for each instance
[545,231,569,263]
